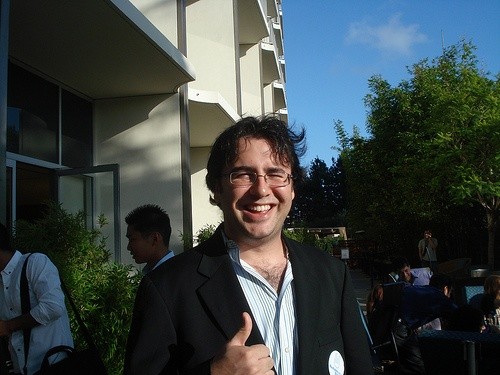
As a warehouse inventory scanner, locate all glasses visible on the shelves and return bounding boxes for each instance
[217,169,297,188]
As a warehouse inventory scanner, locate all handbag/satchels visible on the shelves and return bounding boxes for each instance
[32,345,107,375]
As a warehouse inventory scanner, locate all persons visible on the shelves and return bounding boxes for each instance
[0,220,74,375]
[367,287,383,314]
[125,204,176,275]
[418,223,440,273]
[399,270,468,330]
[124,111,374,375]
[480,274,500,325]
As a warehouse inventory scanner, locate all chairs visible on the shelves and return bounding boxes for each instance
[357,271,500,375]
